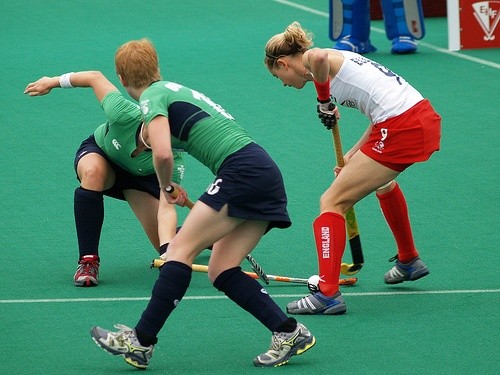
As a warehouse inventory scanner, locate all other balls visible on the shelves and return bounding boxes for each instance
[307,274,321,291]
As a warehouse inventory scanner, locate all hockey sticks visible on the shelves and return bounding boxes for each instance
[329,98,359,279]
[149,256,357,285]
[165,184,269,285]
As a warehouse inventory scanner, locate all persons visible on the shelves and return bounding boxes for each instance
[89,38,317,369]
[24,70,185,286]
[327,0,426,54]
[264,21,441,314]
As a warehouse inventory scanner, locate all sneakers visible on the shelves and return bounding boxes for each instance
[286,283,347,315]
[73,254,100,286]
[90,324,154,368]
[253,322,315,367]
[383,254,428,284]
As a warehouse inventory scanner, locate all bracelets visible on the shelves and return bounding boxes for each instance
[60,71,75,89]
[159,185,167,189]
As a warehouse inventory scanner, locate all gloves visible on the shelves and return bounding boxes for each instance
[315,95,341,130]
